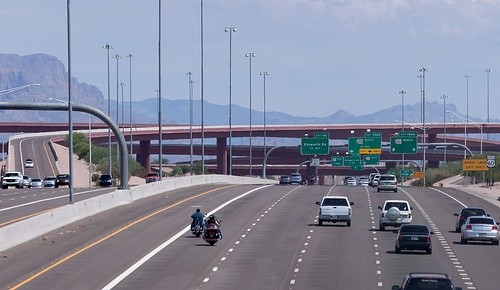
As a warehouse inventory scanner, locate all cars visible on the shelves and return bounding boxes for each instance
[30,178,43,187]
[454,208,490,233]
[316,196,354,227]
[278,173,312,186]
[393,224,434,254]
[343,173,381,188]
[26,158,33,168]
[392,271,462,290]
[461,216,500,245]
[22,175,31,188]
[44,176,56,187]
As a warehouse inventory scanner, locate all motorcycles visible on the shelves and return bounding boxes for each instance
[202,219,223,246]
[190,212,207,237]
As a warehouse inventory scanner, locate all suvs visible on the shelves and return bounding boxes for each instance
[377,174,398,192]
[99,175,112,187]
[55,174,71,188]
[378,200,413,231]
[0,172,23,189]
[146,172,160,183]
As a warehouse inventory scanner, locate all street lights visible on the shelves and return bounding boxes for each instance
[245,52,256,177]
[112,52,124,162]
[224,25,237,174]
[416,75,422,123]
[419,66,428,188]
[485,68,491,140]
[464,74,471,124]
[127,50,134,157]
[102,42,115,175]
[399,90,407,169]
[260,71,270,179]
[440,94,449,161]
[118,81,127,124]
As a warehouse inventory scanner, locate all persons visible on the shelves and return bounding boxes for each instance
[204,215,222,240]
[191,209,206,231]
